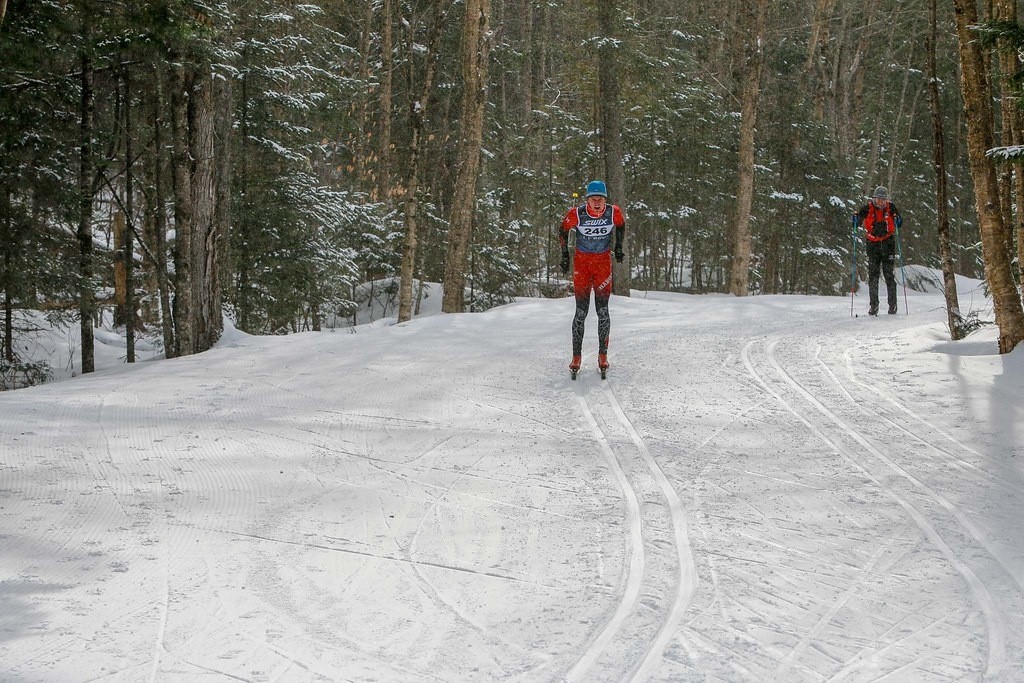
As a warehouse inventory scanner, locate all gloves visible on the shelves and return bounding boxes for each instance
[615,247,624,262]
[560,257,571,274]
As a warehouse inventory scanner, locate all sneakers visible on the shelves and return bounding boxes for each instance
[888,304,897,314]
[569,355,581,369]
[598,354,610,368]
[868,305,879,315]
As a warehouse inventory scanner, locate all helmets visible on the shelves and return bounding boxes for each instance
[586,181,607,197]
[873,186,888,199]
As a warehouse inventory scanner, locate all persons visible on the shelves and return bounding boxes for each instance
[851,186,903,315]
[559,181,624,380]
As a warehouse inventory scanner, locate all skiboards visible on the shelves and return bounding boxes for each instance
[569,366,608,381]
